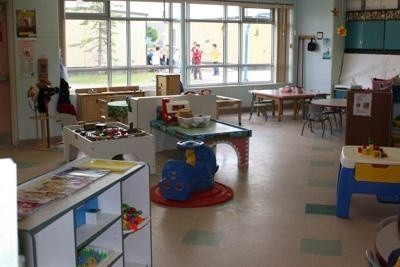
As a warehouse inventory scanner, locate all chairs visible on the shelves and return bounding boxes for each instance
[322,106,343,129]
[248,92,273,119]
[301,105,333,138]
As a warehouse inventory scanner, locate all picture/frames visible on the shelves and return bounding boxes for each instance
[317,31,323,38]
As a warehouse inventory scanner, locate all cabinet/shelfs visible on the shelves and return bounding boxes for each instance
[75,86,145,124]
[128,93,216,129]
[334,89,347,112]
[346,86,393,145]
[156,74,180,95]
[18,153,153,267]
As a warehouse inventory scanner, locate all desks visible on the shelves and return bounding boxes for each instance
[151,118,253,169]
[362,215,400,267]
[107,100,129,121]
[297,91,330,113]
[216,98,243,125]
[63,122,156,175]
[248,89,314,121]
[311,99,347,127]
[336,146,400,218]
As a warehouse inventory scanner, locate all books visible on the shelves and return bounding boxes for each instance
[17,169,112,221]
[72,159,137,172]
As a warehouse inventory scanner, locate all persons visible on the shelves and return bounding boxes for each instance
[148,48,154,65]
[210,43,220,75]
[152,46,160,82]
[190,42,197,73]
[193,44,203,80]
